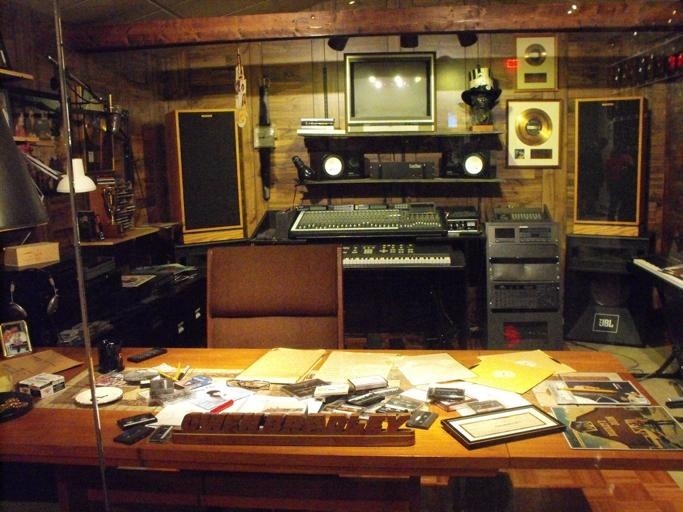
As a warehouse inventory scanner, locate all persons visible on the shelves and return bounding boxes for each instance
[469,92,491,126]
[605,139,637,221]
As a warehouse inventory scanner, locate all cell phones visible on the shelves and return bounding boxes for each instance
[150,425,173,442]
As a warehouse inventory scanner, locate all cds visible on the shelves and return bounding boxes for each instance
[124,368,159,382]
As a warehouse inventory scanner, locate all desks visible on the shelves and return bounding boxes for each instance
[80,227,158,246]
[1,346,682,511]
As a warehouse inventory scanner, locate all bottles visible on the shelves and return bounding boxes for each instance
[14,109,49,139]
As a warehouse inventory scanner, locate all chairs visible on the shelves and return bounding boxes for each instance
[205,244,345,349]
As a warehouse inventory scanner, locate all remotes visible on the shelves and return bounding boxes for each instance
[128,347,167,363]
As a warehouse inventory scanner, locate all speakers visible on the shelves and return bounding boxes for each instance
[442,143,490,179]
[573,96,648,237]
[164,108,245,245]
[310,144,364,181]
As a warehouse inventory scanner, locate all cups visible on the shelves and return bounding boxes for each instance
[96,333,125,375]
[75,210,122,239]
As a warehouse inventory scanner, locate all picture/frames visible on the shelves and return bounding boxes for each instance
[0,320,35,357]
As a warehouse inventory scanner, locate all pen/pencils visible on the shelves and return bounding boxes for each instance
[211,401,233,413]
[158,362,190,381]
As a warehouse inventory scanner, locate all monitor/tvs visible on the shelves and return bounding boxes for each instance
[346,54,435,132]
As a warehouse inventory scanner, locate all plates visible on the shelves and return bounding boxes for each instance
[76,387,124,406]
[0,392,32,422]
